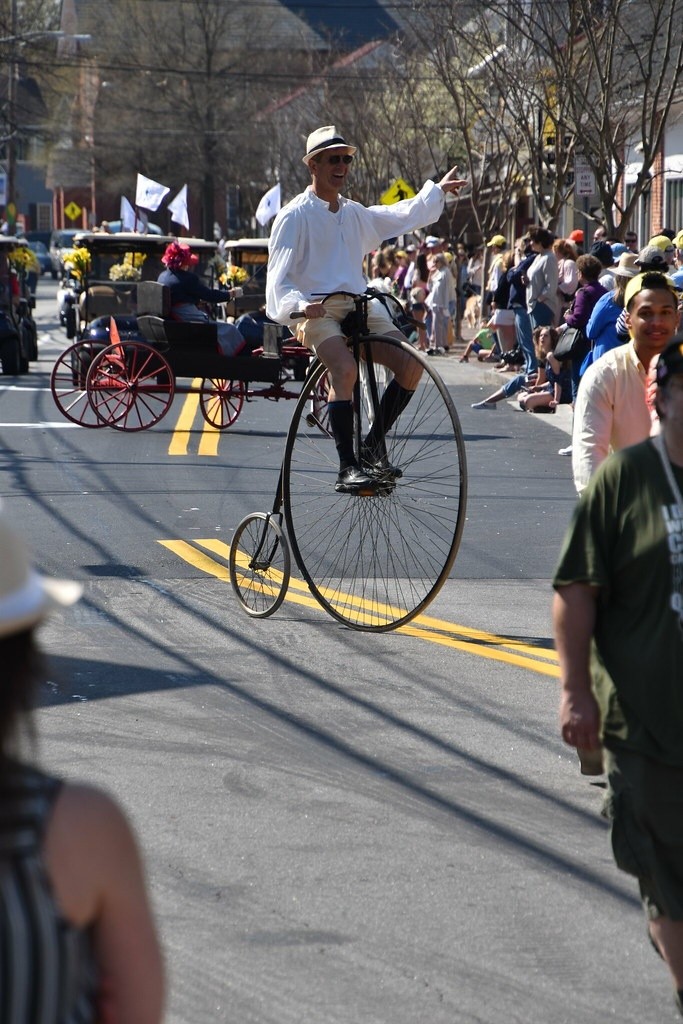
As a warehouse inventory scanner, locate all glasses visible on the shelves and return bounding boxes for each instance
[329,154,354,164]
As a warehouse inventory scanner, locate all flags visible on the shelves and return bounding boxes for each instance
[120,196,143,233]
[167,184,189,231]
[255,184,280,226]
[136,173,170,212]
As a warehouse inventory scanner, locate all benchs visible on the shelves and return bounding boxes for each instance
[136,281,217,351]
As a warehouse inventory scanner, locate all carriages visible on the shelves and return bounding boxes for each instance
[50,275,406,435]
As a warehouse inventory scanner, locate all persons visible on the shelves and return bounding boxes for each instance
[552,330,683,1017]
[157,242,246,356]
[264,125,468,493]
[460,226,683,501]
[0,523,166,1024]
[363,230,469,354]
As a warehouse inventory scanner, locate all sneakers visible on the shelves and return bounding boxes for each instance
[335,468,381,493]
[472,400,497,409]
[360,443,403,477]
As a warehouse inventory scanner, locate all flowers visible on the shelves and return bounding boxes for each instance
[108,263,140,283]
[218,266,249,300]
[163,242,185,272]
[62,247,92,283]
[9,247,40,290]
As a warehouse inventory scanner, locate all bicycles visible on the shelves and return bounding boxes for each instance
[228,287,469,632]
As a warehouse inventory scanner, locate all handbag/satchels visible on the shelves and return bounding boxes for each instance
[558,280,582,302]
[554,327,586,362]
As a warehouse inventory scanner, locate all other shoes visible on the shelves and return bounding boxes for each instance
[459,356,514,372]
[558,445,573,457]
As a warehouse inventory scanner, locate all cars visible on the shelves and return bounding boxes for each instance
[0,228,308,381]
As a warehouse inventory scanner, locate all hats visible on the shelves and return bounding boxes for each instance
[161,243,197,266]
[487,235,507,247]
[0,503,81,634]
[624,271,680,339]
[397,236,454,262]
[647,235,675,251]
[590,241,615,265]
[567,229,584,244]
[607,252,641,277]
[672,230,683,249]
[637,247,667,265]
[608,243,631,263]
[302,126,357,165]
